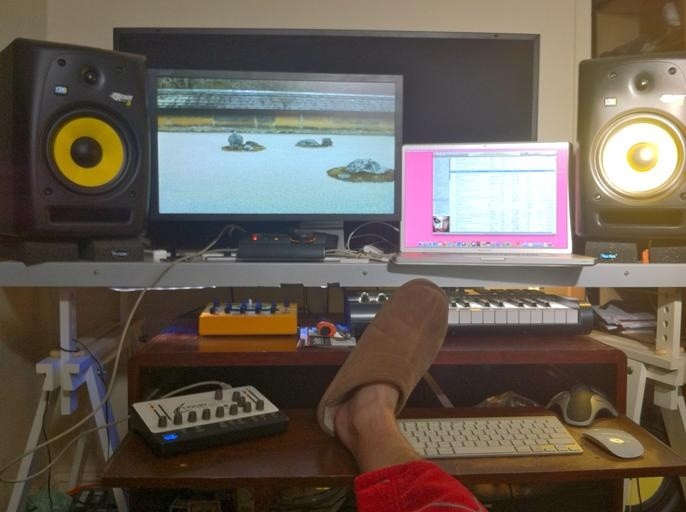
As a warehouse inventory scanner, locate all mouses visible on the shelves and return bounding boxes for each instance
[582,427,645,459]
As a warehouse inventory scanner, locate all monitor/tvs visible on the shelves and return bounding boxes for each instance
[148,68,403,261]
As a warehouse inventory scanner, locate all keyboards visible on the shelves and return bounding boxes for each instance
[397,416,583,460]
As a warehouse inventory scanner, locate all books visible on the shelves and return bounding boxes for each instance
[592,302,658,334]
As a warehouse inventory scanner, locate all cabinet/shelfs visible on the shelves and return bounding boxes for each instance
[95,312,686,511]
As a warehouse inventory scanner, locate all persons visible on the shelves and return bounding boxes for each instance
[432,216,451,233]
[315,277,491,512]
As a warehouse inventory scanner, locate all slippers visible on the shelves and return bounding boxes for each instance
[315,279,449,435]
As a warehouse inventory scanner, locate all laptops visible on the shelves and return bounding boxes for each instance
[397,142,597,266]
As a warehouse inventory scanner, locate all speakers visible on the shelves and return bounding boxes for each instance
[0,35,151,266]
[576,50,685,242]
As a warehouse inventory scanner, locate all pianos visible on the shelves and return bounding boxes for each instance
[343,288,594,338]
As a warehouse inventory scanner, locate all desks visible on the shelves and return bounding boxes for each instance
[0,245,686,512]
